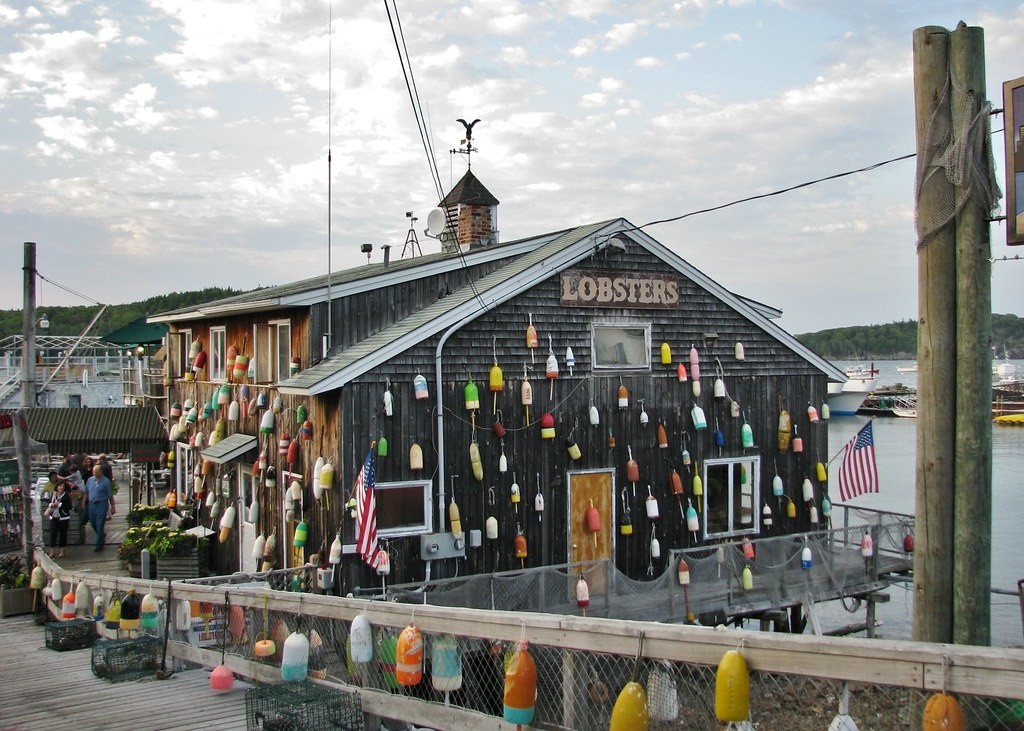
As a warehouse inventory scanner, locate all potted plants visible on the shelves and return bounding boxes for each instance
[0,553,36,619]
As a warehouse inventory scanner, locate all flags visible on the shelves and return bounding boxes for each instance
[355,448,378,569]
[838,421,878,501]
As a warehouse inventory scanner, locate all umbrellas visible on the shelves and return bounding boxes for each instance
[100,312,170,372]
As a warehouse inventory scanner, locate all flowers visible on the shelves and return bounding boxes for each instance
[117,522,200,556]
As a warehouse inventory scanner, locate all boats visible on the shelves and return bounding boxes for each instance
[897,361,918,372]
[827,351,879,415]
[992,343,1024,401]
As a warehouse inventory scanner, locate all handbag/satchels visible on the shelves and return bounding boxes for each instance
[111,478,120,495]
[44,491,57,517]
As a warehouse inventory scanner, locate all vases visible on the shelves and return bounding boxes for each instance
[127,548,210,580]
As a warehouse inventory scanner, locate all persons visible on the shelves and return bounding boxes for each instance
[41,453,94,499]
[49,482,72,559]
[97,453,112,520]
[56,464,85,490]
[83,465,116,552]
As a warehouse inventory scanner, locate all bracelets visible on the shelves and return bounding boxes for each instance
[113,505,115,507]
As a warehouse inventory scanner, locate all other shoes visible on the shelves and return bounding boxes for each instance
[101,534,106,545]
[94,547,103,552]
[57,552,65,558]
[49,553,55,558]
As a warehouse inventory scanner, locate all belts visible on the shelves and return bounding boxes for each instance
[90,499,108,504]
